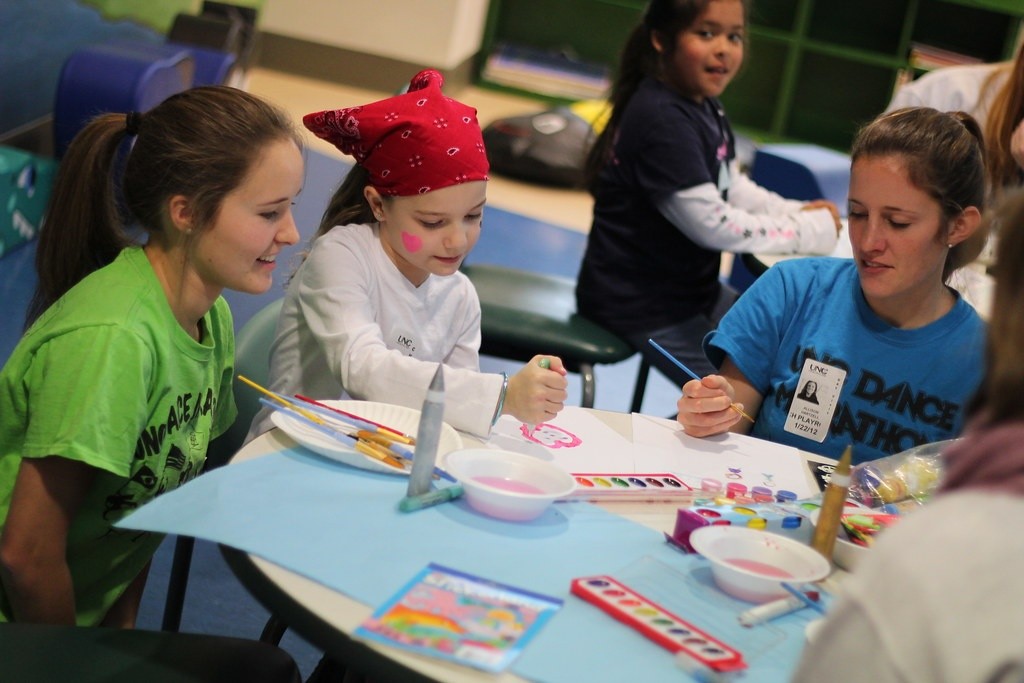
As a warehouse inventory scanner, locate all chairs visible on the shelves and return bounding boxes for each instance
[459,260,654,412]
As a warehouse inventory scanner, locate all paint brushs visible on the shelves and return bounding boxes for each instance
[780,579,832,619]
[236,371,459,486]
[646,337,758,424]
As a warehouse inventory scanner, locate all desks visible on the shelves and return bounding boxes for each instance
[222,399,912,683]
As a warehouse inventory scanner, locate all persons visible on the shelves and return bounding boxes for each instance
[783,185,1024,683]
[669,103,992,479]
[873,23,1024,303]
[569,1,848,443]
[228,67,568,454]
[0,83,312,620]
[0,614,303,682]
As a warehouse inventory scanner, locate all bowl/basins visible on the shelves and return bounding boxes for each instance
[689,525,832,605]
[809,507,871,569]
[442,448,577,522]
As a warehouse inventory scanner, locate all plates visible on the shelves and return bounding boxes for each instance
[270,399,462,475]
